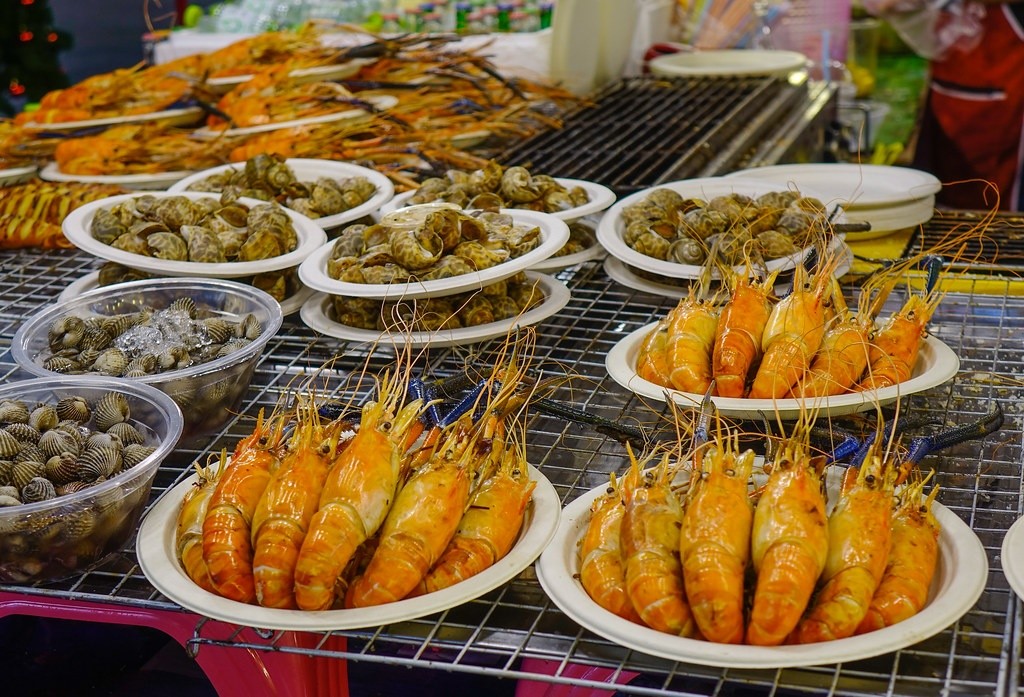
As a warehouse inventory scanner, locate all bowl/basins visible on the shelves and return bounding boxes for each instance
[10,277,284,437]
[0,375,185,586]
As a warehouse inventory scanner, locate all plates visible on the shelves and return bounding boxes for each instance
[371,177,614,224]
[525,219,606,276]
[298,209,570,301]
[649,49,808,78]
[535,459,991,670]
[1001,513,1024,609]
[723,161,943,243]
[604,316,961,421]
[604,252,854,304]
[135,437,562,633]
[596,177,848,279]
[60,189,328,277]
[165,158,396,230]
[0,65,489,190]
[299,287,572,349]
[58,270,318,318]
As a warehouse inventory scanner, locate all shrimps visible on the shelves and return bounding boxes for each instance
[0,20,600,250]
[174,177,1024,645]
